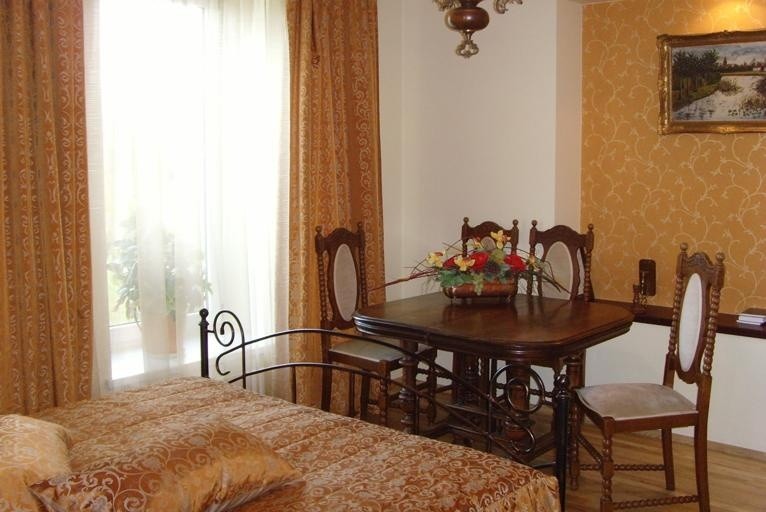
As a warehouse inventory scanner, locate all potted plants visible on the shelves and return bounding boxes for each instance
[107,220,213,356]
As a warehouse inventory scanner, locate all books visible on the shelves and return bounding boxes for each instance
[735,308,766,326]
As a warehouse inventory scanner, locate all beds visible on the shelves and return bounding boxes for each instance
[0,308,570,512]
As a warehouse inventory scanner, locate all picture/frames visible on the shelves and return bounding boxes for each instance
[657,29,766,135]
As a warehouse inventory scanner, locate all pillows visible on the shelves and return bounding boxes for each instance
[29,414,304,512]
[0,414,72,512]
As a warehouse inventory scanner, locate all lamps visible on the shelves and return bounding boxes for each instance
[434,0,523,58]
